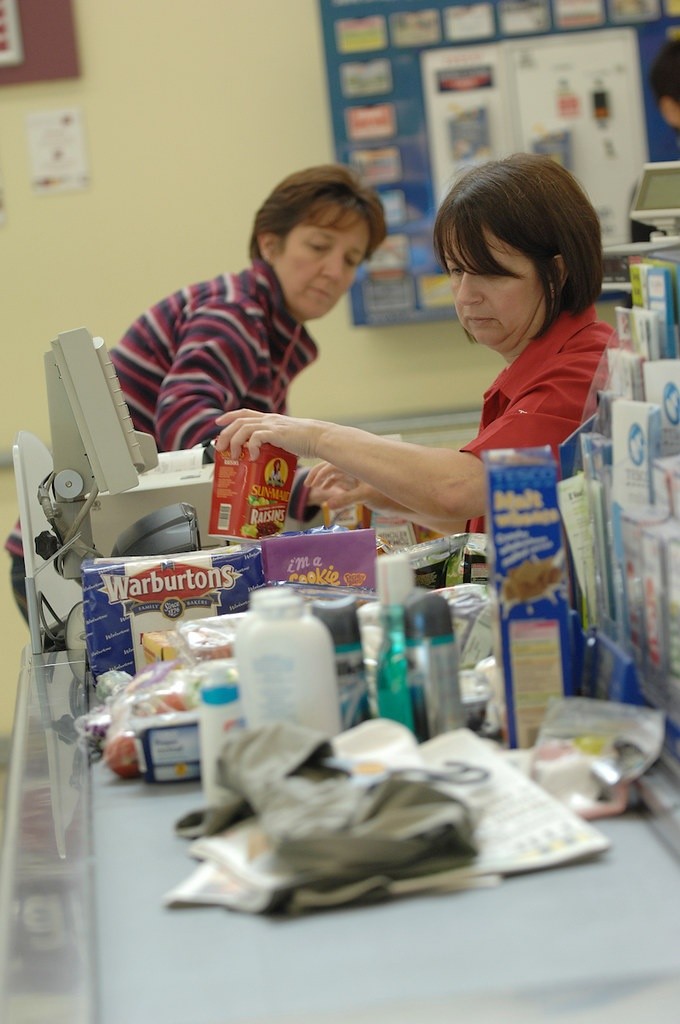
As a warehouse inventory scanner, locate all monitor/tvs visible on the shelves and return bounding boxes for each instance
[629,161,680,220]
[41,326,160,504]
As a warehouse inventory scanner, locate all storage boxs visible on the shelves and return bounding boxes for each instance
[207,436,297,542]
[80,544,263,686]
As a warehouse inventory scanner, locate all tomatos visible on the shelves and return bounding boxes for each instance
[107,734,143,777]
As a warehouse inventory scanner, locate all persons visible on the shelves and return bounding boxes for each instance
[217,151,639,538]
[108,161,389,522]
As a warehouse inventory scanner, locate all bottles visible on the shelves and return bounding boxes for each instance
[199,515,487,828]
